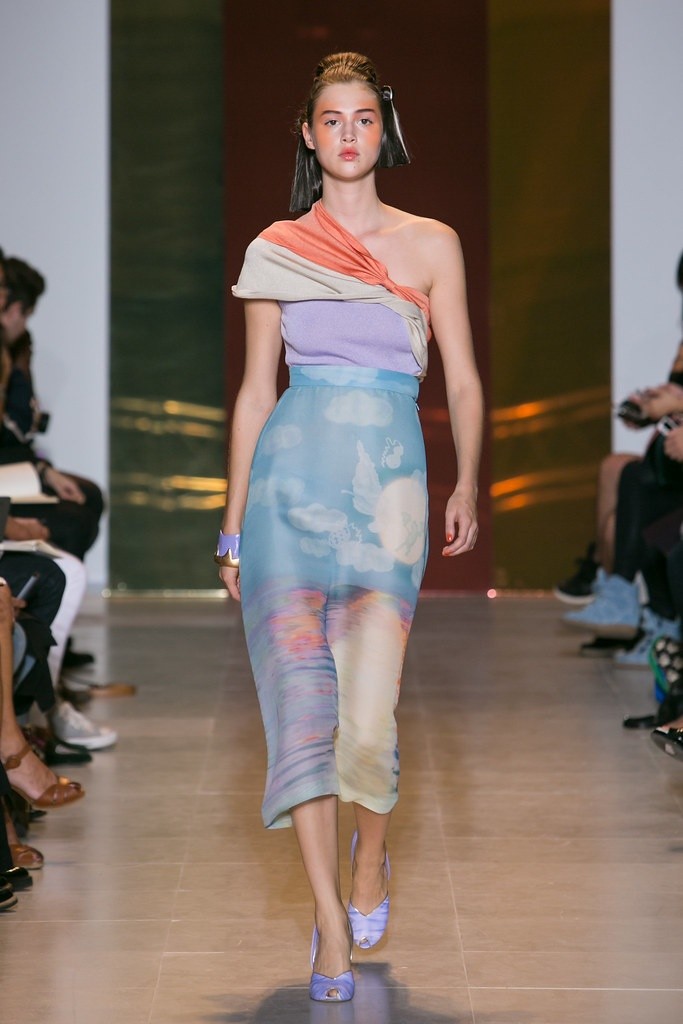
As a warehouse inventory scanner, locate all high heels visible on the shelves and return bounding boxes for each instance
[310,914,355,1002]
[348,829,390,950]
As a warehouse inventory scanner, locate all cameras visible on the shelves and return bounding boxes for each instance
[617,399,658,430]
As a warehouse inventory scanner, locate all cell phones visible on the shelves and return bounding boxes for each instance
[656,417,678,438]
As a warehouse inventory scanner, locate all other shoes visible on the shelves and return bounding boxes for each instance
[552,538,683,759]
[0,637,120,910]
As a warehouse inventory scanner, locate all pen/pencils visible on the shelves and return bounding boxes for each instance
[16,572,39,600]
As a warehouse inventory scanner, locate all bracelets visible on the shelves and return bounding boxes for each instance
[217,529,240,559]
[218,553,240,568]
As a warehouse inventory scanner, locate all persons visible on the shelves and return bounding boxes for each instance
[1,247,119,914]
[218,52,485,1004]
[552,250,683,763]
